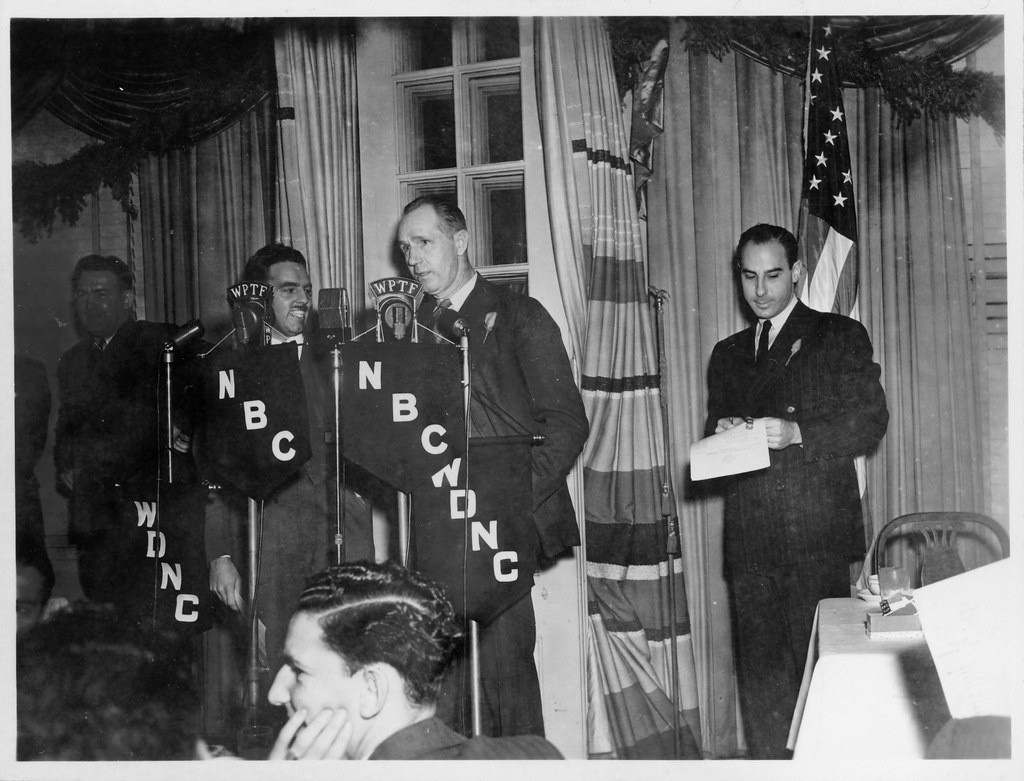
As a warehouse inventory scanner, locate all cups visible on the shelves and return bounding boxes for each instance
[866,574,883,595]
[878,565,911,603]
[207,745,224,754]
[235,725,274,760]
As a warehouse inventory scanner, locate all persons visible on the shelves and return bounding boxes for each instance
[397,195,592,738]
[268,561,566,759]
[16,253,211,761]
[706,224,889,760]
[198,242,372,748]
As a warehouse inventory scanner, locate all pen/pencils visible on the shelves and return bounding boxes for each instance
[728,416,734,426]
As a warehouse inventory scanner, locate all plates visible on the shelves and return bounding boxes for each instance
[857,587,894,602]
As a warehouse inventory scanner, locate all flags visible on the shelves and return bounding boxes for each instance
[806,15,868,500]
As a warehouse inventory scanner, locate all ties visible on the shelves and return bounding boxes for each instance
[92,337,106,361]
[757,319,773,370]
[429,298,452,334]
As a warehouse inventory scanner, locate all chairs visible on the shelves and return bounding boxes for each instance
[875,512,1010,589]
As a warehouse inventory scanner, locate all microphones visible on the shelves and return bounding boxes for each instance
[168,321,207,348]
[317,287,350,348]
[438,309,470,337]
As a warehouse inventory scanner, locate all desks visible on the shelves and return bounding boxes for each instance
[785,598,1011,760]
